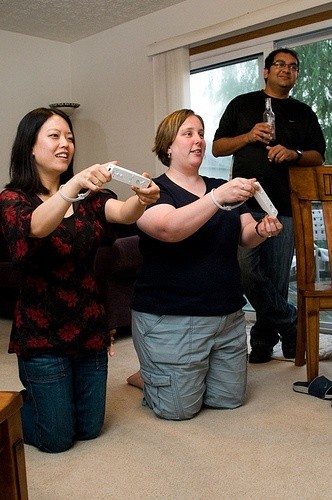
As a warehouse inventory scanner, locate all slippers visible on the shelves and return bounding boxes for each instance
[293,375,332,400]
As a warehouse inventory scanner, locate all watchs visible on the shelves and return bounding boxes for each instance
[295,148,302,163]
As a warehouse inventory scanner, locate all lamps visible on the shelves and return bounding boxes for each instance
[49,102,80,118]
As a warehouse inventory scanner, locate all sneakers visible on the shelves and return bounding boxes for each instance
[249,346,272,363]
[279,321,298,359]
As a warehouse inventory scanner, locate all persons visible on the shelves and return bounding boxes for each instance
[127,109,282,422]
[1,107,163,452]
[210,49,325,364]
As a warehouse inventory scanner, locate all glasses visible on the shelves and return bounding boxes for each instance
[272,60,299,72]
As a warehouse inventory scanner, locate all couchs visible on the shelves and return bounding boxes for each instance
[111,221,140,338]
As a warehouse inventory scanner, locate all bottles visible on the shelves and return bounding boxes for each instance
[262,98,277,139]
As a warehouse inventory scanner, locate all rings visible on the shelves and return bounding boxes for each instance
[94,179,99,186]
[242,182,246,190]
[267,234,272,237]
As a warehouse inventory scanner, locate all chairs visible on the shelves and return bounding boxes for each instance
[288,162,332,385]
[0,390,29,499]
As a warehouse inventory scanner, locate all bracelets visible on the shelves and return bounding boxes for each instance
[254,219,263,238]
